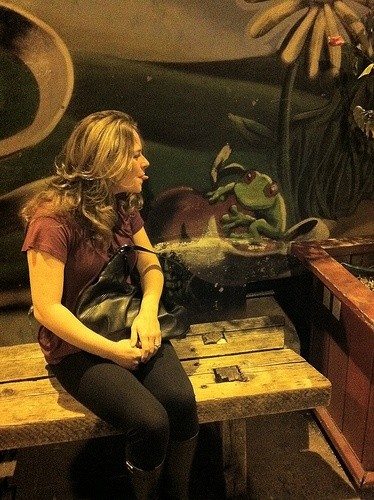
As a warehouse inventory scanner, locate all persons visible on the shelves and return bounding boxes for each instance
[19,110,199,500]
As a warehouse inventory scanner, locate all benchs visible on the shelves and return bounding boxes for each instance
[0,315,287,384]
[0,349,332,500]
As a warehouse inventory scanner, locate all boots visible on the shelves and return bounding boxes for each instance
[166,431,200,500]
[126,453,166,500]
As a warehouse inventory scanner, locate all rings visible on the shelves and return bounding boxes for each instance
[155,343,161,349]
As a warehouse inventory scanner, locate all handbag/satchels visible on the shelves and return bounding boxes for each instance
[74,244,196,342]
